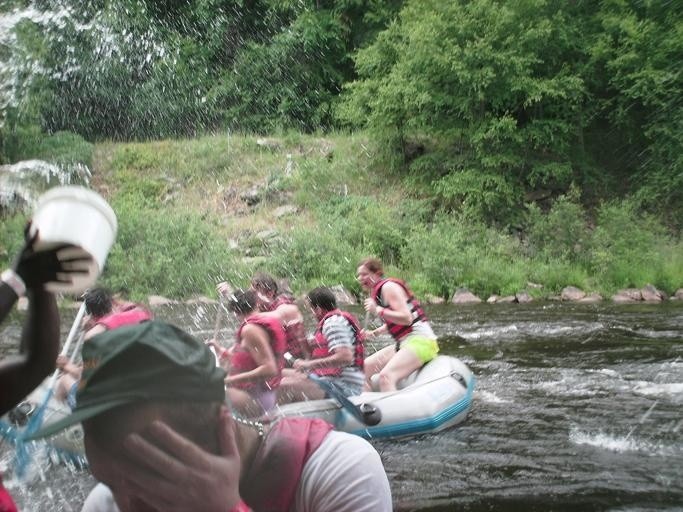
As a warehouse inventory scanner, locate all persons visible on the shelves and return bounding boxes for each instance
[114,288,148,321]
[278,284,365,401]
[48,286,136,373]
[0,219,93,511]
[25,321,392,511]
[215,272,309,376]
[348,258,438,392]
[204,292,287,416]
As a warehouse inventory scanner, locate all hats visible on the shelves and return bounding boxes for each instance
[23,319,226,442]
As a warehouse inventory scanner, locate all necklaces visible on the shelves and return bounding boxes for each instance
[233,416,265,441]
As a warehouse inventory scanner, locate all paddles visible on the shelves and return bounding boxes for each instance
[12,297,86,478]
[284,350,366,425]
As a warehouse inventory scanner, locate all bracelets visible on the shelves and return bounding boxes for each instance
[1,267,27,299]
[378,307,387,318]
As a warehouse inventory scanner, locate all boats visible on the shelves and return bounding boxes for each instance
[0,354,476,466]
